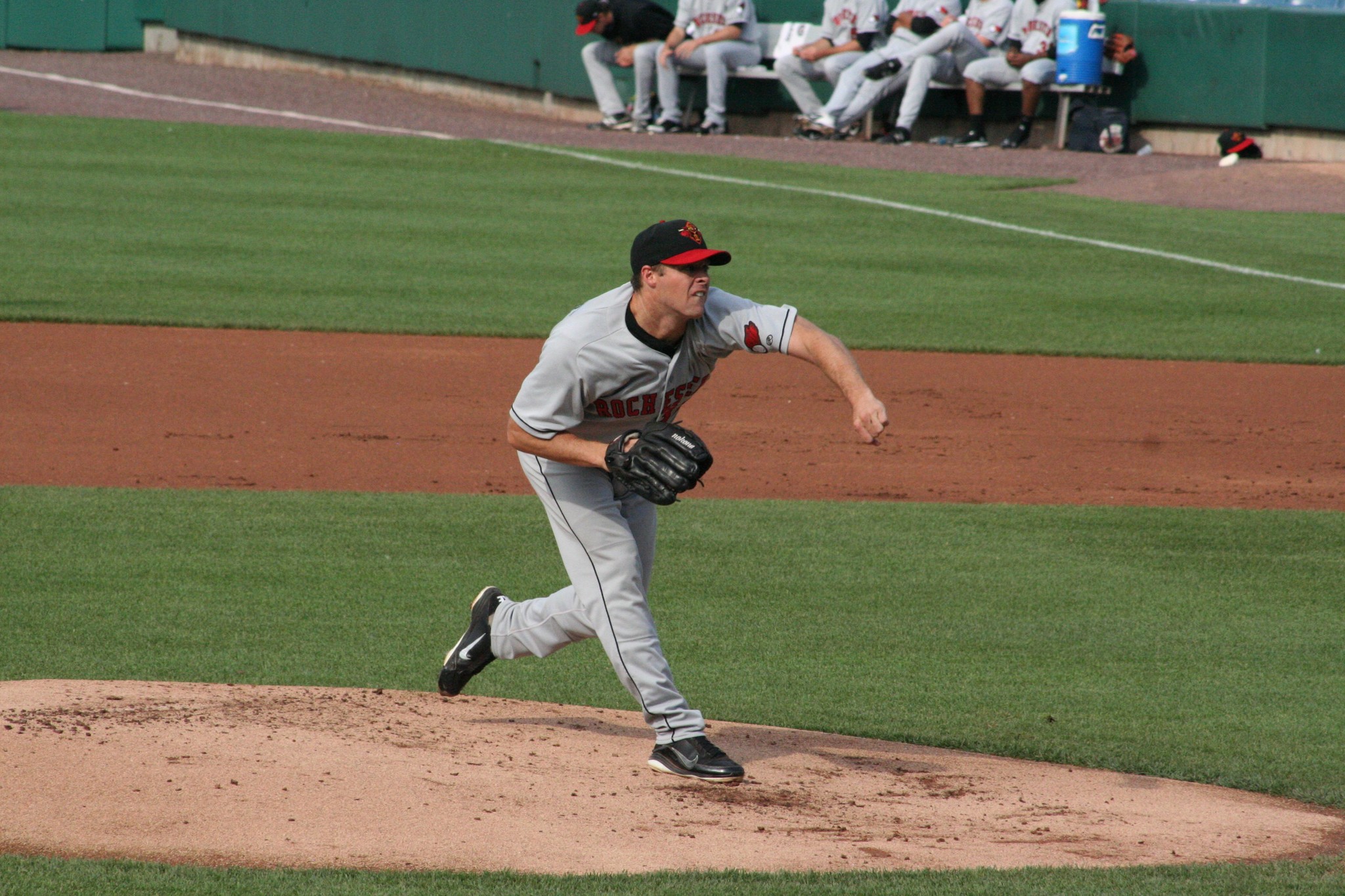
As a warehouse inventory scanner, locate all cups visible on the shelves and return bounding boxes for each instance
[1137,144,1152,156]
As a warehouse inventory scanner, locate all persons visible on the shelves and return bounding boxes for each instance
[438,219,891,783]
[575,0,694,134]
[645,0,762,136]
[772,0,1100,152]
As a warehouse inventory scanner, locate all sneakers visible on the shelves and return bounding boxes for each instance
[1001,125,1030,148]
[586,113,632,130]
[802,131,846,142]
[863,58,899,80]
[878,127,912,145]
[843,120,862,140]
[438,585,509,697]
[628,118,652,133]
[793,114,835,134]
[648,736,745,782]
[953,130,989,148]
[646,117,682,134]
[693,121,728,135]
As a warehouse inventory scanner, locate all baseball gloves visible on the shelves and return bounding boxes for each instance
[602,416,713,505]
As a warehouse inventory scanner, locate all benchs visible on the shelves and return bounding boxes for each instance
[673,19,1124,150]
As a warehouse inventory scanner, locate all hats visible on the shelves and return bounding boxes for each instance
[575,0,601,36]
[1217,129,1254,152]
[630,219,731,274]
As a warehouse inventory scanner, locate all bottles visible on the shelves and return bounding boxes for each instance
[929,136,954,145]
[1219,153,1238,166]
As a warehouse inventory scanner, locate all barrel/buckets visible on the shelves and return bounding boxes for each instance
[1056,9,1107,86]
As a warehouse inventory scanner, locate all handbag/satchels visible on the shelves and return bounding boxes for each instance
[1067,107,1130,154]
